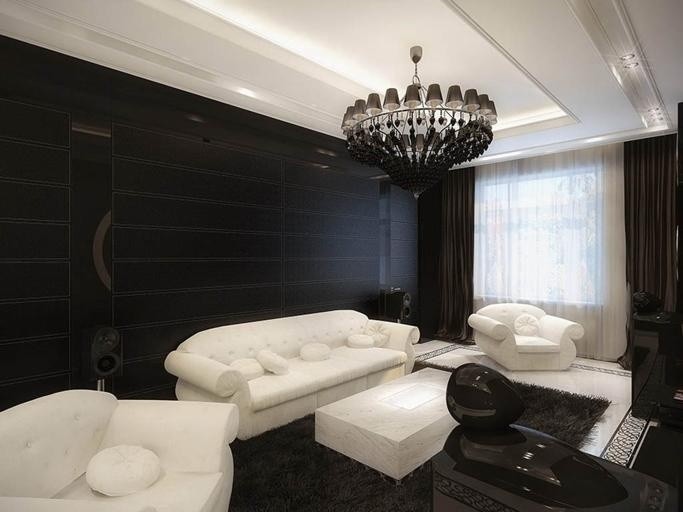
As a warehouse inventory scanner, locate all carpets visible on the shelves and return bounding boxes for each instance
[231,360,612,511]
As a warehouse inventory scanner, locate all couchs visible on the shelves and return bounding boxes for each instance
[164,310,418,440]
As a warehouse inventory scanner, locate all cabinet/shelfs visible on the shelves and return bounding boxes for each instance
[630,310,682,421]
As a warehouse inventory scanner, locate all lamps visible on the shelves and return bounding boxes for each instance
[340,45,501,198]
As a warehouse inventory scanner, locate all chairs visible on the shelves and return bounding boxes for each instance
[0,388,240,510]
[467,302,587,373]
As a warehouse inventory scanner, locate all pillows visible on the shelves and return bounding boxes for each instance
[83,445,164,500]
[299,342,334,362]
[256,350,290,374]
[514,314,541,337]
[345,334,376,348]
[230,359,263,380]
